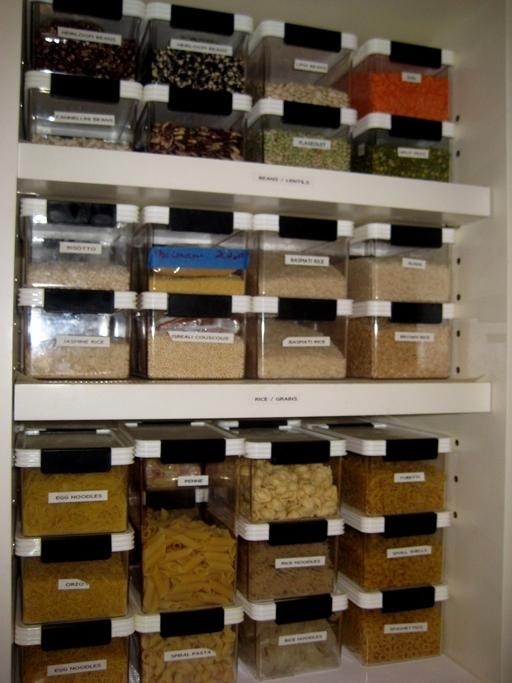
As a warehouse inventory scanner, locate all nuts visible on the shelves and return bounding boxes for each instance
[19,461,451,683]
[27,18,452,183]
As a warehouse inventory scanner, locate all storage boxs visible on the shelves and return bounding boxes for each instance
[349,575,449,667]
[238,516,347,602]
[335,508,452,592]
[12,534,136,622]
[128,204,251,295]
[15,286,141,384]
[234,432,348,523]
[26,63,138,153]
[348,220,459,300]
[22,0,145,77]
[351,111,456,184]
[249,290,353,381]
[17,199,145,290]
[321,38,456,114]
[238,586,345,682]
[348,302,459,381]
[128,599,244,682]
[14,426,135,537]
[136,75,251,161]
[117,421,240,484]
[341,426,454,513]
[131,486,241,614]
[244,98,358,170]
[12,614,132,683]
[251,15,353,106]
[137,1,250,92]
[248,216,356,299]
[133,291,254,383]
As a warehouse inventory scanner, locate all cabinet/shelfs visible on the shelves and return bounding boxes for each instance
[0,2,509,680]
